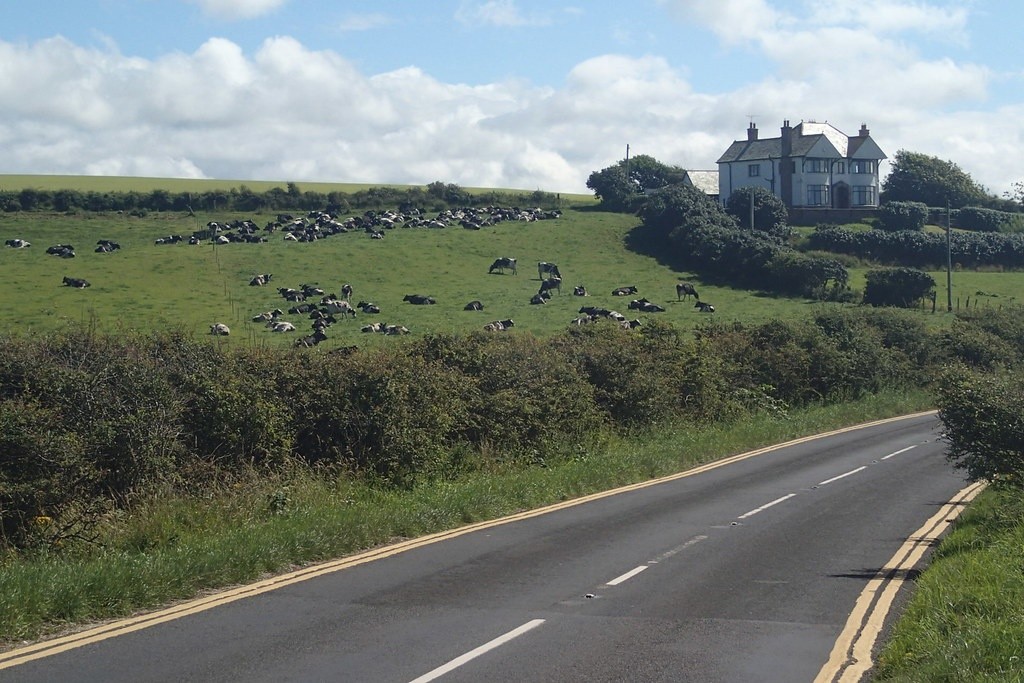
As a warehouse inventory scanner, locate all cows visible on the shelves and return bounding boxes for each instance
[4,239,32,250]
[95,239,122,253]
[45,243,75,260]
[571,285,667,332]
[488,256,519,278]
[248,272,436,364]
[482,318,515,334]
[572,285,591,296]
[62,276,91,290]
[209,323,230,337]
[528,261,563,306]
[155,203,564,245]
[675,283,715,313]
[462,300,484,312]
